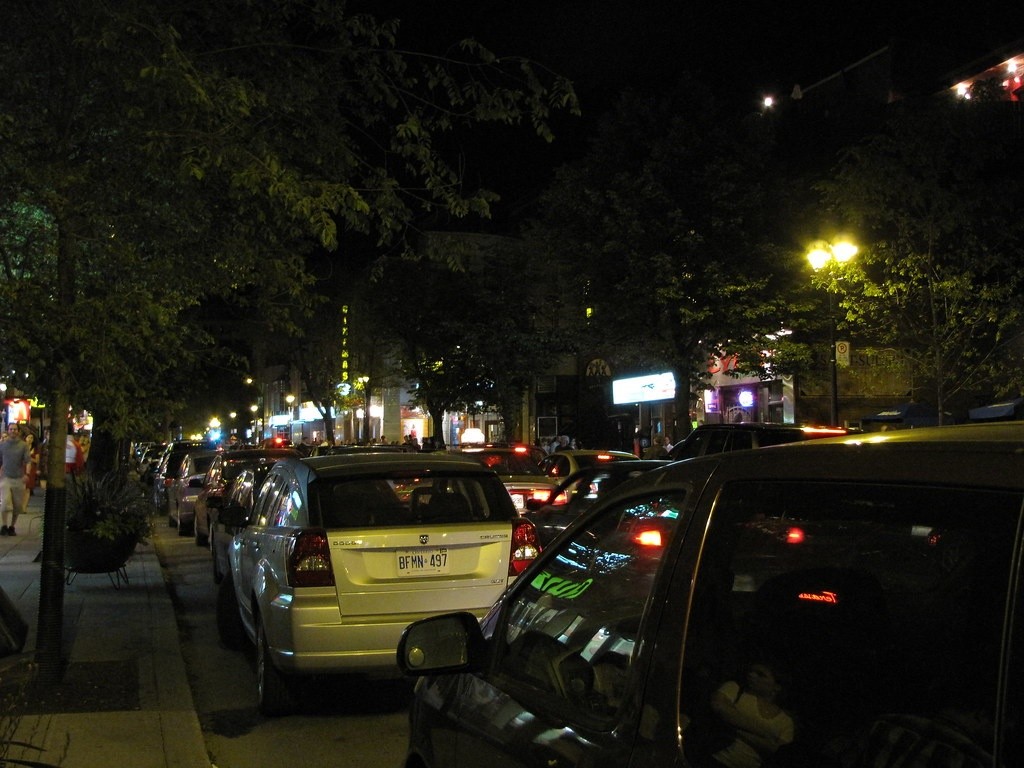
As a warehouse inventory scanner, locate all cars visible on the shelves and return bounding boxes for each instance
[137,442,417,588]
[397,422,1024,768]
[227,453,542,710]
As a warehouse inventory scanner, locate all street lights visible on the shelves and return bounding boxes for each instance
[230,412,236,441]
[808,242,857,427]
[285,395,296,445]
[251,405,258,449]
[358,375,370,442]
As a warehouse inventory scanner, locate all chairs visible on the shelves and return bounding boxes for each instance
[410,487,441,522]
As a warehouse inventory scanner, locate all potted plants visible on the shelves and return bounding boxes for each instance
[29,468,154,574]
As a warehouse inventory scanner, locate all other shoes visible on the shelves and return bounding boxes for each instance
[8,526,16,536]
[0,526,8,535]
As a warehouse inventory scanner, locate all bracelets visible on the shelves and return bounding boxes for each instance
[25,473,30,476]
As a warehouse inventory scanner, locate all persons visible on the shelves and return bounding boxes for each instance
[0,423,91,536]
[533,434,583,453]
[298,435,444,457]
[642,434,675,460]
